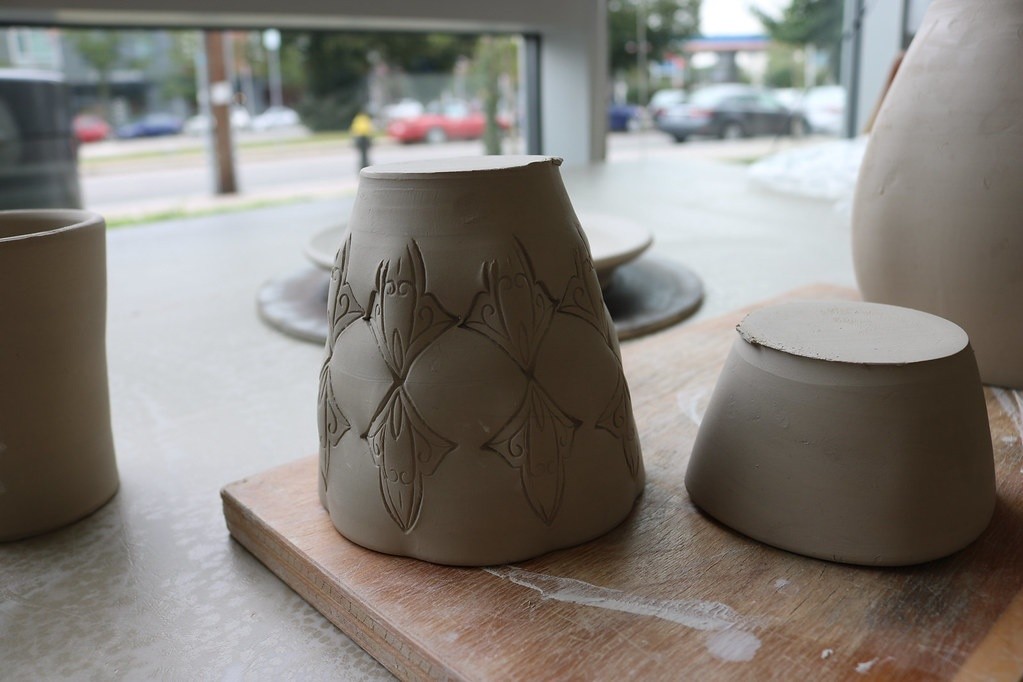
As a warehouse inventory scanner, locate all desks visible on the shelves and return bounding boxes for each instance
[0,156,856,682]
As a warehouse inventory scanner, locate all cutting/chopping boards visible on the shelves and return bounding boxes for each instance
[216,284,1023,682]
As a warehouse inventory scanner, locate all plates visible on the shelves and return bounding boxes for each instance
[306,211,655,288]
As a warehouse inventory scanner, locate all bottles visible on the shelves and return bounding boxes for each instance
[850,1,1023,389]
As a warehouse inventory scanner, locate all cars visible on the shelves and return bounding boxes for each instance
[389,98,511,144]
[607,82,811,144]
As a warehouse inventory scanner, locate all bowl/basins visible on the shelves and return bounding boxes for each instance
[681,302,1000,566]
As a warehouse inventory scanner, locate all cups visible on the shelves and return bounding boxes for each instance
[317,154,648,567]
[1,208,120,543]
